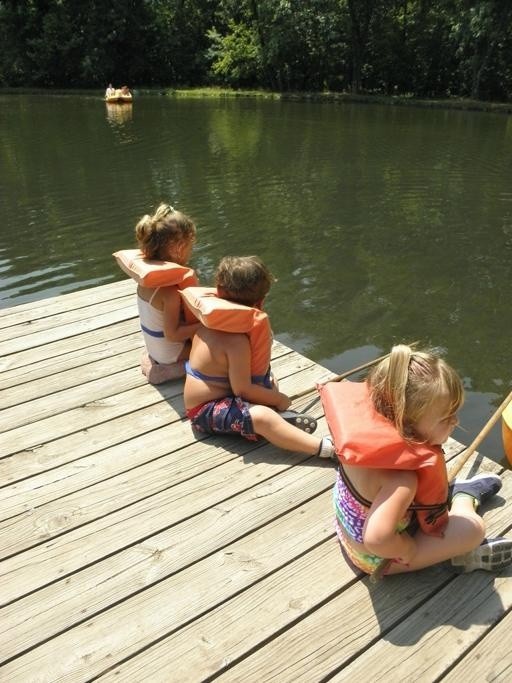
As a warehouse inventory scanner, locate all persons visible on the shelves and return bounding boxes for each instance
[111,202,203,385]
[314,344,512,583]
[178,253,337,462]
[106,82,116,97]
[121,84,129,94]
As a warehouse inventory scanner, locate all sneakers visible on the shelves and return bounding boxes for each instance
[446,474,501,510]
[139,348,187,385]
[277,409,318,434]
[443,536,512,574]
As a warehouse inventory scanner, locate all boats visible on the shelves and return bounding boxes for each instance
[104,88,133,102]
[105,101,134,122]
